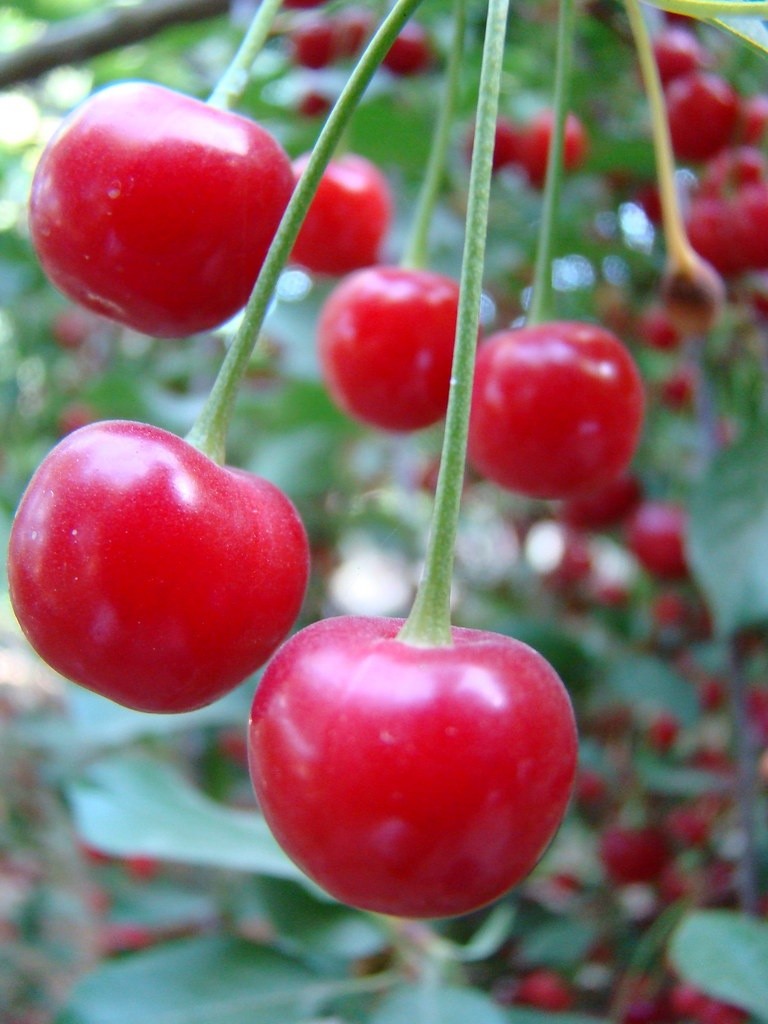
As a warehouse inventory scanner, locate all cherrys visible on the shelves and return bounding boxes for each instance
[4,0,768,1024]
